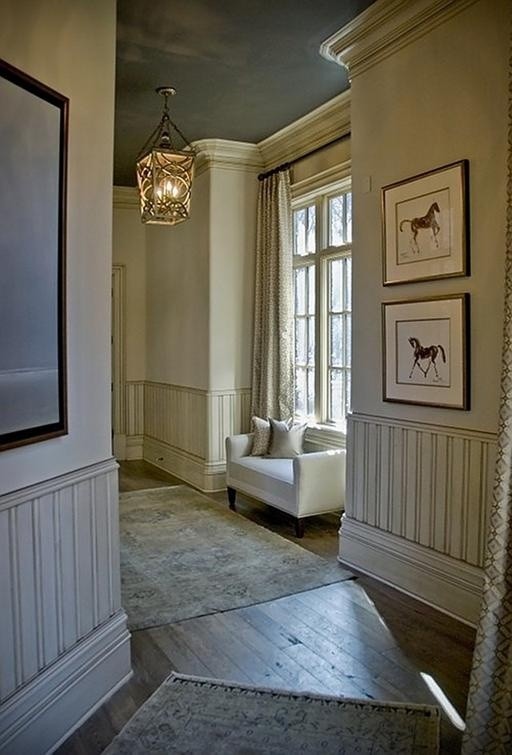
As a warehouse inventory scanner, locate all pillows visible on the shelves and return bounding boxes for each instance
[250,415,308,458]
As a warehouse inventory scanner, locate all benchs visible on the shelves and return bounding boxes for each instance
[225,433,347,538]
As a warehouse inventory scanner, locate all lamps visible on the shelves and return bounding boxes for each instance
[135,88,197,226]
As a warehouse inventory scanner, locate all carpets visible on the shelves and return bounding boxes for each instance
[118,483,359,632]
[100,670,442,755]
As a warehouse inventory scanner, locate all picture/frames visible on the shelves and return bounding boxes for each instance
[0,59,70,450]
[381,158,471,411]
